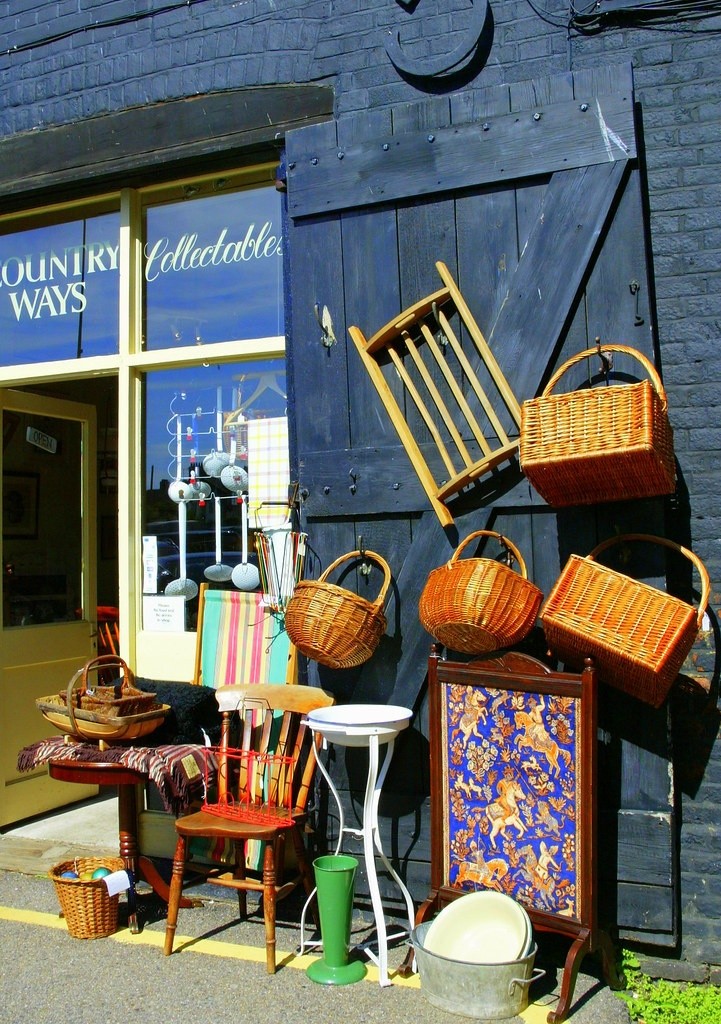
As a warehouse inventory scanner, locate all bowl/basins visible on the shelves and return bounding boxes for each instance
[309,701,414,746]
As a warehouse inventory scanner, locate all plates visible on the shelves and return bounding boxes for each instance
[424,891,532,964]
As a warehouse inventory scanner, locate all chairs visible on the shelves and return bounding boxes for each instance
[163,684,337,974]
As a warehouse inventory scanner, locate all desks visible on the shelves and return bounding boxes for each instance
[48,758,204,934]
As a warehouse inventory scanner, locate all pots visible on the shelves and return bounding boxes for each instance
[407,919,547,1021]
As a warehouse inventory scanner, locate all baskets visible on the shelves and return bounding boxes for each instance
[518,345,677,508]
[36,663,171,742]
[59,663,157,718]
[284,550,390,670]
[538,533,710,708]
[48,857,124,940]
[417,529,545,656]
